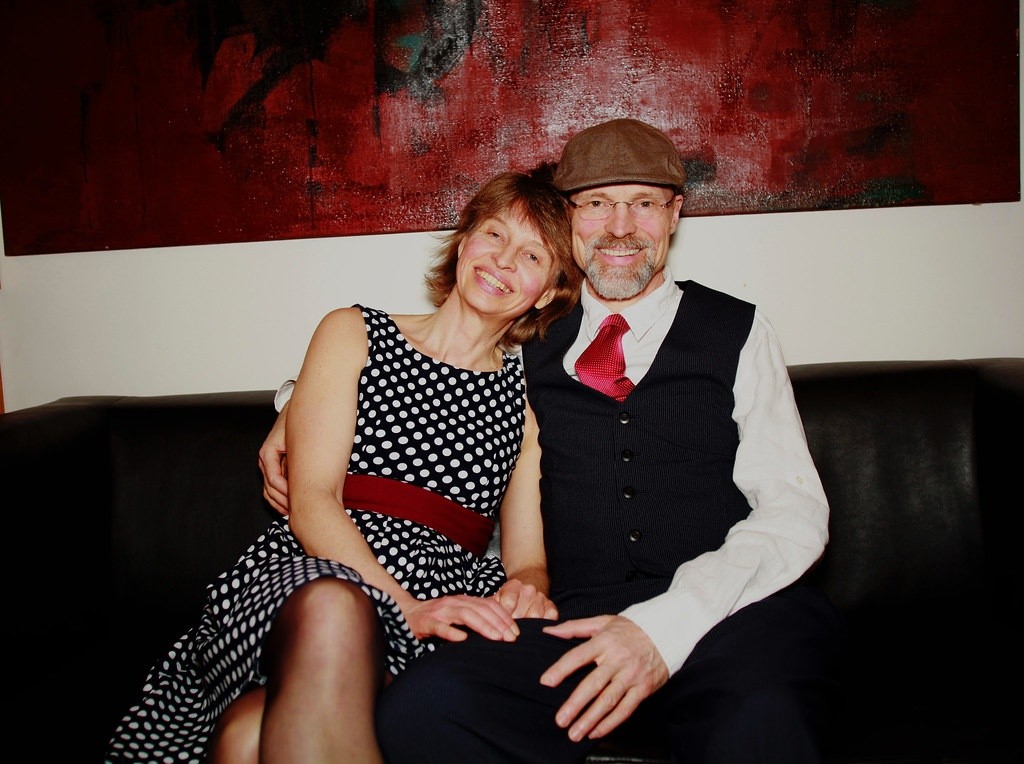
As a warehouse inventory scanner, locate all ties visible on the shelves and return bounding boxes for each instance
[573,313,637,402]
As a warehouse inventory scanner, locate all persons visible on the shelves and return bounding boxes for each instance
[255,119,835,764]
[104,161,583,764]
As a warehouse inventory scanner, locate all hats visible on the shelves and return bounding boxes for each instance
[552,119,686,193]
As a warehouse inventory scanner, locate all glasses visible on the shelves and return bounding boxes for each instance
[566,193,676,220]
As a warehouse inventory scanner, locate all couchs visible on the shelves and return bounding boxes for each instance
[0,356,1024,764]
